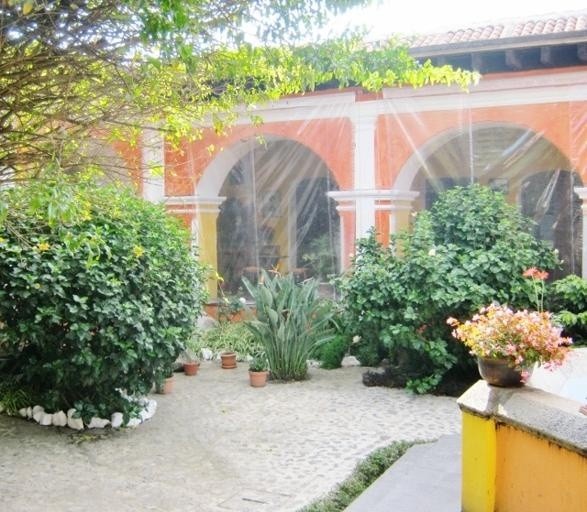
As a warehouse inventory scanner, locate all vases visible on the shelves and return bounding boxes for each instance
[477,355,531,385]
[221,352,237,369]
[182,361,200,375]
[155,377,173,394]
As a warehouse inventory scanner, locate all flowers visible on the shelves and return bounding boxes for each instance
[443,267,575,386]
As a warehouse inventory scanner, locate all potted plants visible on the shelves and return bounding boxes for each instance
[247,356,269,388]
